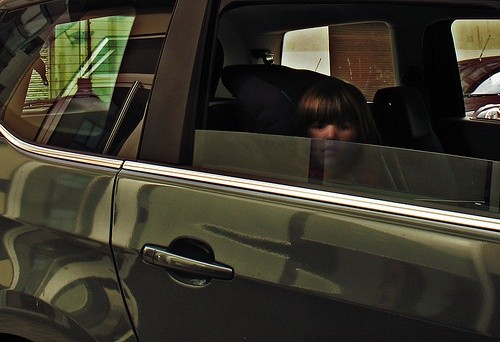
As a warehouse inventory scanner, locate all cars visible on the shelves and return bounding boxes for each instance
[0,0,500,342]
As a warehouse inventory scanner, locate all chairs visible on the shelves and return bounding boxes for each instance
[205,63,487,206]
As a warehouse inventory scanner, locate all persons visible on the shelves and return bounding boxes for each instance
[295,79,379,186]
